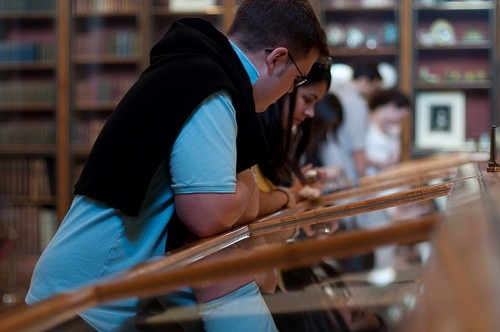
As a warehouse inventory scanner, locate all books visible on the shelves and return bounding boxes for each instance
[0,7,143,304]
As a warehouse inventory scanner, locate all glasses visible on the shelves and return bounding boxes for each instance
[265,47,308,87]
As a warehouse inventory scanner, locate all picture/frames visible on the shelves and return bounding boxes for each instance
[414,92,466,150]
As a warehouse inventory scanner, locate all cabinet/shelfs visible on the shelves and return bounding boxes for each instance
[0,0,500,310]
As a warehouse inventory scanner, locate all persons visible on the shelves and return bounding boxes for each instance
[331,183,440,233]
[282,264,390,332]
[24,1,411,332]
[197,280,281,332]
[366,237,431,280]
